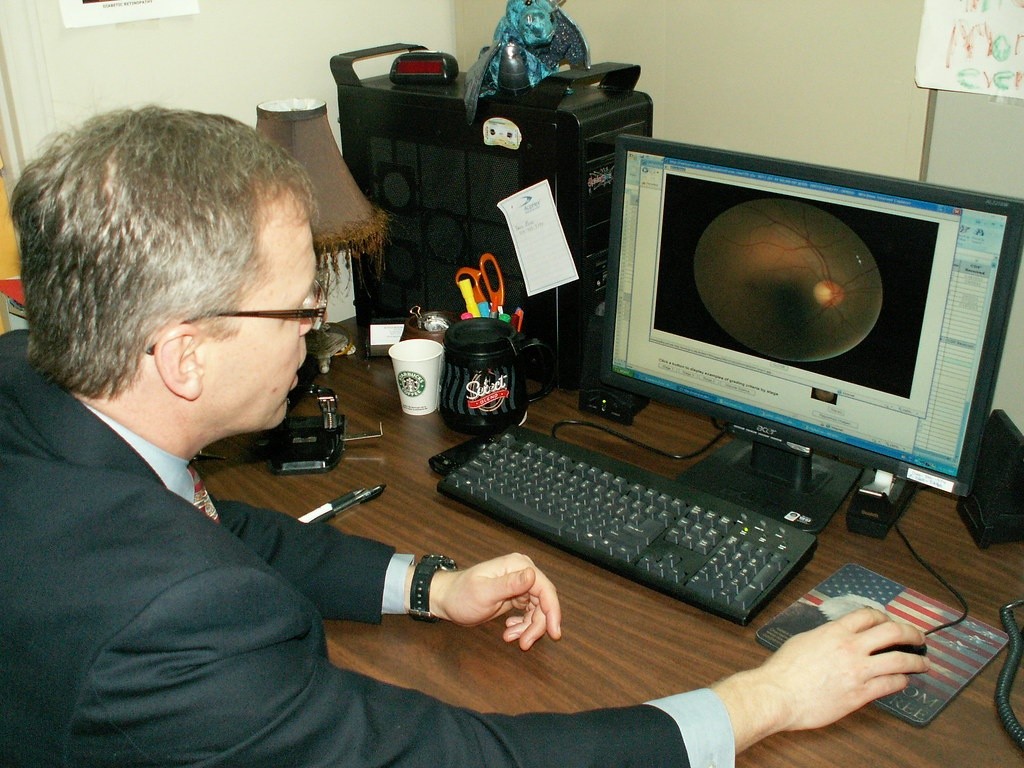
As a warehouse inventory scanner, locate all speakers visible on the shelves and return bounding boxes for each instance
[578,301,651,423]
[956,409,1022,550]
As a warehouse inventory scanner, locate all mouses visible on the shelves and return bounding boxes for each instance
[871,639,930,658]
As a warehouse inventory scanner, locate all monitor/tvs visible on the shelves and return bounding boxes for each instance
[596,136,1023,497]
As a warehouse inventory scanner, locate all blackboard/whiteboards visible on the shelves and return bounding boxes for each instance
[917,91,1024,438]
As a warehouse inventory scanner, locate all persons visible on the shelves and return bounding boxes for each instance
[0,101,932,768]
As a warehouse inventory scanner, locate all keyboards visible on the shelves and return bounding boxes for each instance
[438,425,817,626]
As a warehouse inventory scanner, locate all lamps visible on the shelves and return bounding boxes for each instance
[256,96,394,374]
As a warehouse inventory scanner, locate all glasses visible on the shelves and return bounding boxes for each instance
[143,277,328,357]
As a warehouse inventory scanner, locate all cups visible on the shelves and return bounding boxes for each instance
[437,317,559,435]
[388,339,444,415]
[404,309,458,345]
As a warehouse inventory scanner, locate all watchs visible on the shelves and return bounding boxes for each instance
[405,553,457,622]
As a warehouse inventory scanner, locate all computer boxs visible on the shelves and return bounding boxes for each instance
[330,45,653,391]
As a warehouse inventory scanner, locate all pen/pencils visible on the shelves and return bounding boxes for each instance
[296,487,369,523]
[305,483,387,525]
[511,307,525,334]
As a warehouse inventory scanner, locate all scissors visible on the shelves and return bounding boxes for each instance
[454,253,505,319]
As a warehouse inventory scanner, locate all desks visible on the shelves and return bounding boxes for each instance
[189,311,1024,768]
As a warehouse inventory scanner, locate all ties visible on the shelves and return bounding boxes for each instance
[189,467,222,525]
